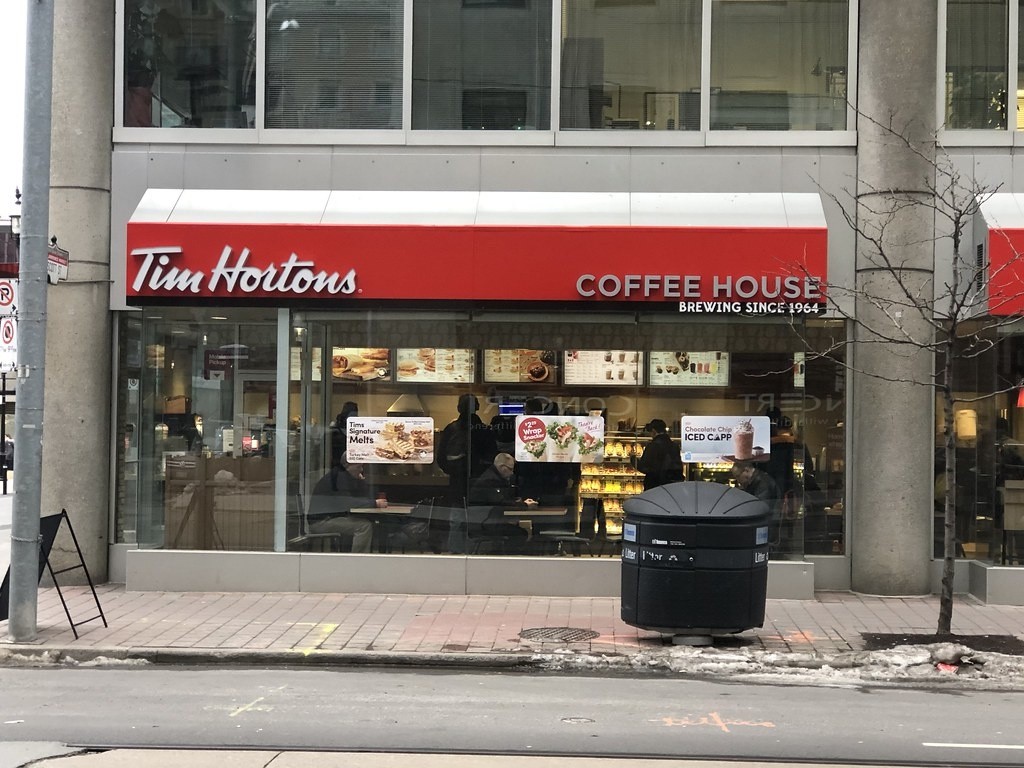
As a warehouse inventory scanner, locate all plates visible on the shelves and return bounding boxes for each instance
[527,362,548,381]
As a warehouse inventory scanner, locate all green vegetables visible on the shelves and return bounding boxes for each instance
[523,422,604,458]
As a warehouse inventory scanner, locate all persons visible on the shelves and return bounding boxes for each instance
[4,434,14,470]
[331,401,358,469]
[436,394,497,553]
[307,451,387,554]
[755,405,813,538]
[967,418,1024,479]
[490,399,607,541]
[630,418,685,491]
[731,462,782,541]
[469,452,529,555]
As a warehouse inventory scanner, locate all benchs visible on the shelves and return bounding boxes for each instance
[295,494,341,551]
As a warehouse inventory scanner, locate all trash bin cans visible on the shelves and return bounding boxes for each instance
[621,481,770,635]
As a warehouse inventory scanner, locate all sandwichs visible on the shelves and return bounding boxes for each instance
[374,423,434,459]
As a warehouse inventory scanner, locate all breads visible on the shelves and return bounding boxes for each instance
[582,443,643,532]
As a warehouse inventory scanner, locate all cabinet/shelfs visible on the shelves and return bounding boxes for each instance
[696,446,805,492]
[571,436,689,537]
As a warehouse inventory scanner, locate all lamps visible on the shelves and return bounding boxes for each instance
[292,316,306,329]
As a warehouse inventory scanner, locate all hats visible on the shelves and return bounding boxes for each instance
[458,393,479,412]
[646,419,667,433]
[777,416,792,428]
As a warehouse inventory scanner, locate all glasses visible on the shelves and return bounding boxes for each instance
[500,465,514,472]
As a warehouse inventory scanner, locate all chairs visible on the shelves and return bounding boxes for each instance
[399,496,444,554]
[768,486,800,560]
[551,505,596,557]
[449,496,494,553]
[597,497,623,557]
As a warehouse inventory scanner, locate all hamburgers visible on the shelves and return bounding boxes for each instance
[398,360,420,377]
[419,348,435,372]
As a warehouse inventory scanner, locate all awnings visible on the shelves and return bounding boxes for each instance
[126,187,830,313]
[972,192,1024,317]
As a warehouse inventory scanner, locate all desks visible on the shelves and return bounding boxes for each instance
[351,502,414,553]
[805,508,843,553]
[504,507,567,556]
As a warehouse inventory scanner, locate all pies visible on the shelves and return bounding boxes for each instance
[333,354,363,377]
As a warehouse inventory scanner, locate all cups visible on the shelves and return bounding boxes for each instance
[378,492,385,499]
[634,353,639,363]
[697,363,702,372]
[604,352,611,361]
[633,371,638,380]
[619,353,625,362]
[690,363,695,372]
[618,370,624,378]
[606,370,611,379]
[704,361,718,373]
[752,448,764,457]
[734,432,754,459]
